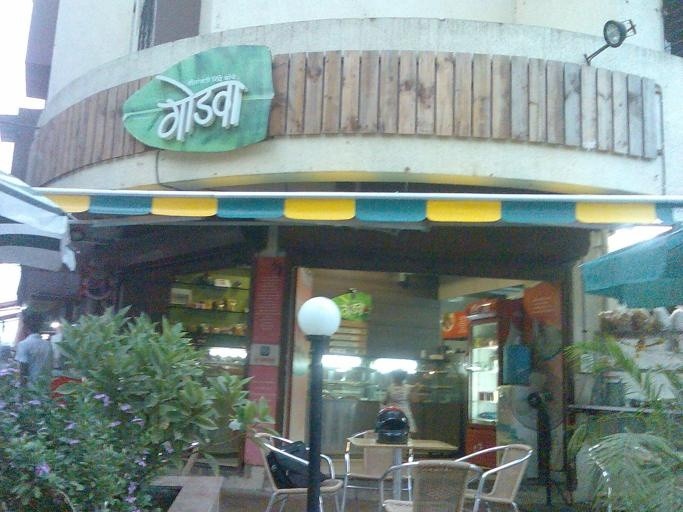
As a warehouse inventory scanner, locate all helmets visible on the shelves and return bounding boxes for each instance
[376,406,409,444]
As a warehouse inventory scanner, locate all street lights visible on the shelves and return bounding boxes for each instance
[297,296,341,512]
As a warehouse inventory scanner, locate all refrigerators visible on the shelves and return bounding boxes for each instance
[465,297,524,466]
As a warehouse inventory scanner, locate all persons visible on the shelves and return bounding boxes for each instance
[381,370,417,439]
[14,308,55,393]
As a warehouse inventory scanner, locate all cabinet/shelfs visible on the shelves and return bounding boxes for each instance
[167,280,251,337]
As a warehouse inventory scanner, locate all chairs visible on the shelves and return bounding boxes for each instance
[253,428,534,512]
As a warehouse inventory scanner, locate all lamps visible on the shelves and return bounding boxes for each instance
[583,19,637,67]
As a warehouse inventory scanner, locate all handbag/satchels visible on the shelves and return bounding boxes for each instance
[266,440,326,489]
